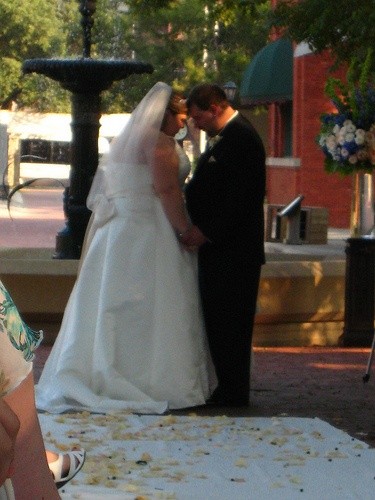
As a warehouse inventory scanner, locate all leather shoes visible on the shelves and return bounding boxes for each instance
[206,395,248,407]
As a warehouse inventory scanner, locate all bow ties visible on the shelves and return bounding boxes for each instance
[206,135,221,142]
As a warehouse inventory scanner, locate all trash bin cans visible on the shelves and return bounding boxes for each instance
[343,236,375,346]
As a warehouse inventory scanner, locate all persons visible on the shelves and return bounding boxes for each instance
[34,81,219,414]
[0,278,87,500]
[185,83,265,406]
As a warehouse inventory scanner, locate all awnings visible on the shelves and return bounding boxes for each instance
[240,36,293,107]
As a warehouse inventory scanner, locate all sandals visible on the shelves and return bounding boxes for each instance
[48,448,86,489]
